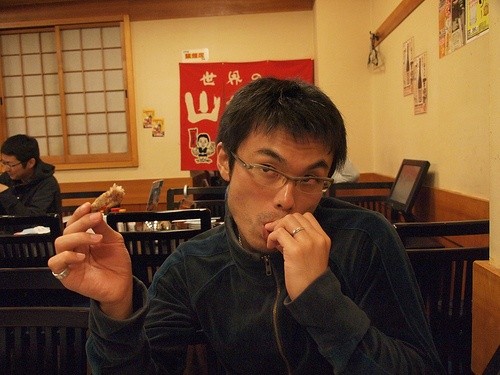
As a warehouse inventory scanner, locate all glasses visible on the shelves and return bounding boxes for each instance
[226,146,334,195]
[1,159,29,170]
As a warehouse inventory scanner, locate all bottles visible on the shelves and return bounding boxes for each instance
[112,207,127,233]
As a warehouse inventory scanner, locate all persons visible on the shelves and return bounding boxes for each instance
[324,151,360,183]
[48,78,446,375]
[0,134,63,232]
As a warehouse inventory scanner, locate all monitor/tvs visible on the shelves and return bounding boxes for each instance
[386,159,431,214]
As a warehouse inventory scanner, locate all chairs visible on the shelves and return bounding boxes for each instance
[0,182,500,375]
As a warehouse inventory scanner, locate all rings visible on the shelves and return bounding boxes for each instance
[52,267,71,280]
[291,226,304,236]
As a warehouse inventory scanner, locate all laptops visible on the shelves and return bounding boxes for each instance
[146,179,163,211]
